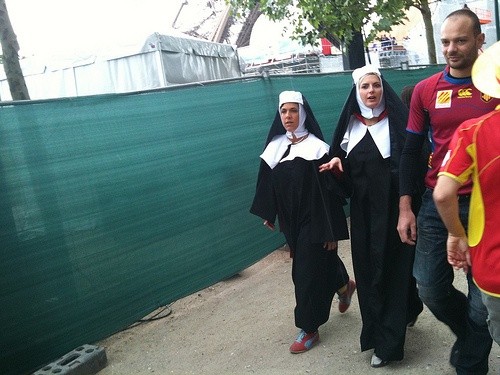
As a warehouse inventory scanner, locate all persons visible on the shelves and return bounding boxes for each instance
[318,64,433,368]
[434,41,500,347]
[248,89,357,354]
[394,8,499,375]
[401,86,417,110]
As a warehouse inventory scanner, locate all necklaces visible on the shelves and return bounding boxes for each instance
[287,135,308,144]
